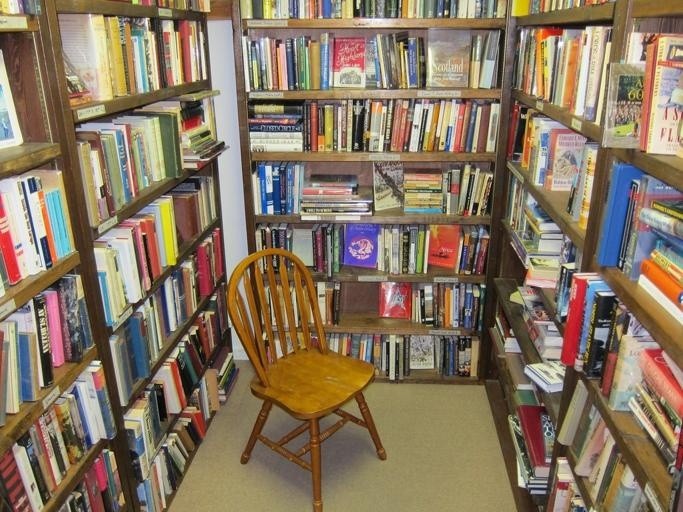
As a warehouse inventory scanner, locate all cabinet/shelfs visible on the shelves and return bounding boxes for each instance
[229,0,519,387]
[1,0,240,510]
[484,1,683,511]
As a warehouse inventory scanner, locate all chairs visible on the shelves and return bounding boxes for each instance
[225,247,387,512]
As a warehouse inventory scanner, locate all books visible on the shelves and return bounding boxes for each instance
[528,0,608,17]
[251,161,494,217]
[267,332,479,381]
[137,350,240,512]
[239,1,509,20]
[75,89,230,225]
[92,175,217,333]
[59,13,209,107]
[248,98,500,154]
[0,47,23,151]
[597,162,683,325]
[0,274,94,437]
[123,283,226,482]
[261,282,340,328]
[130,0,211,12]
[504,174,575,323]
[0,359,118,511]
[599,32,683,157]
[546,456,587,512]
[508,282,566,394]
[0,0,41,15]
[0,168,76,295]
[556,379,666,512]
[58,450,129,511]
[481,311,556,495]
[241,30,502,92]
[506,100,598,232]
[109,227,224,408]
[512,25,614,125]
[376,282,486,331]
[561,271,683,475]
[254,223,490,279]
